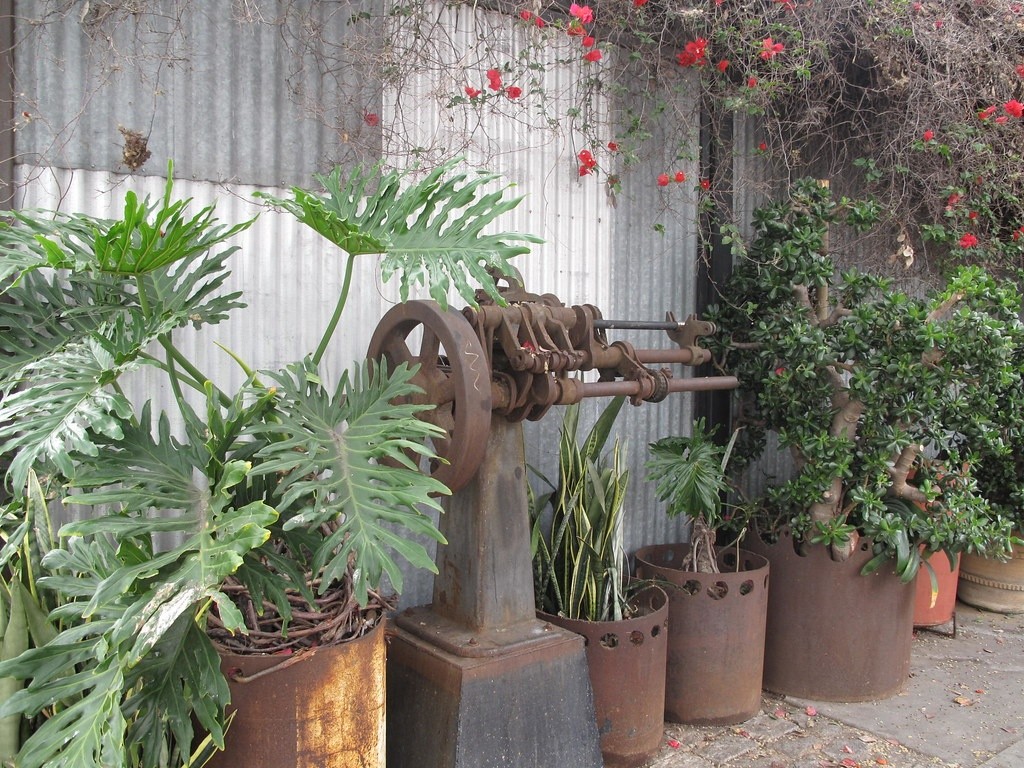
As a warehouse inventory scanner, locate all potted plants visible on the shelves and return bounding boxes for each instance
[518,367,670,768]
[634,415,769,729]
[940,321,1024,614]
[701,174,1024,704]
[1,162,546,768]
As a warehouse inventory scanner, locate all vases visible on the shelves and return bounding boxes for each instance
[899,464,962,626]
